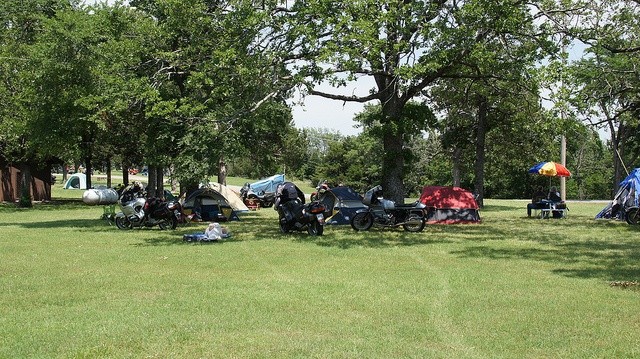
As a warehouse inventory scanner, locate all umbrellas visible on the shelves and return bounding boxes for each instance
[528,161,571,187]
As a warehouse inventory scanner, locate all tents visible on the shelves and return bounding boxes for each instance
[62,172,86,189]
[310,185,367,225]
[363,184,382,205]
[595,167,640,220]
[172,181,250,213]
[417,185,481,225]
[180,188,240,222]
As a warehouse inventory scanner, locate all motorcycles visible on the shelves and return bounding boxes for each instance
[349,186,431,233]
[241,183,276,209]
[275,181,325,237]
[115,180,183,231]
[309,177,330,203]
[624,203,640,225]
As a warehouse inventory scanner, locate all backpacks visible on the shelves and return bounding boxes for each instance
[282,181,298,200]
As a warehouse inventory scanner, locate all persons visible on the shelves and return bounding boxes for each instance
[115,180,143,200]
[547,186,560,199]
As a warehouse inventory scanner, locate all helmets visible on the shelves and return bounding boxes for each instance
[131,181,143,192]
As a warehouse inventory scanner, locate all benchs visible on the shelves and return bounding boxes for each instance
[533,209,547,217]
[542,209,567,219]
